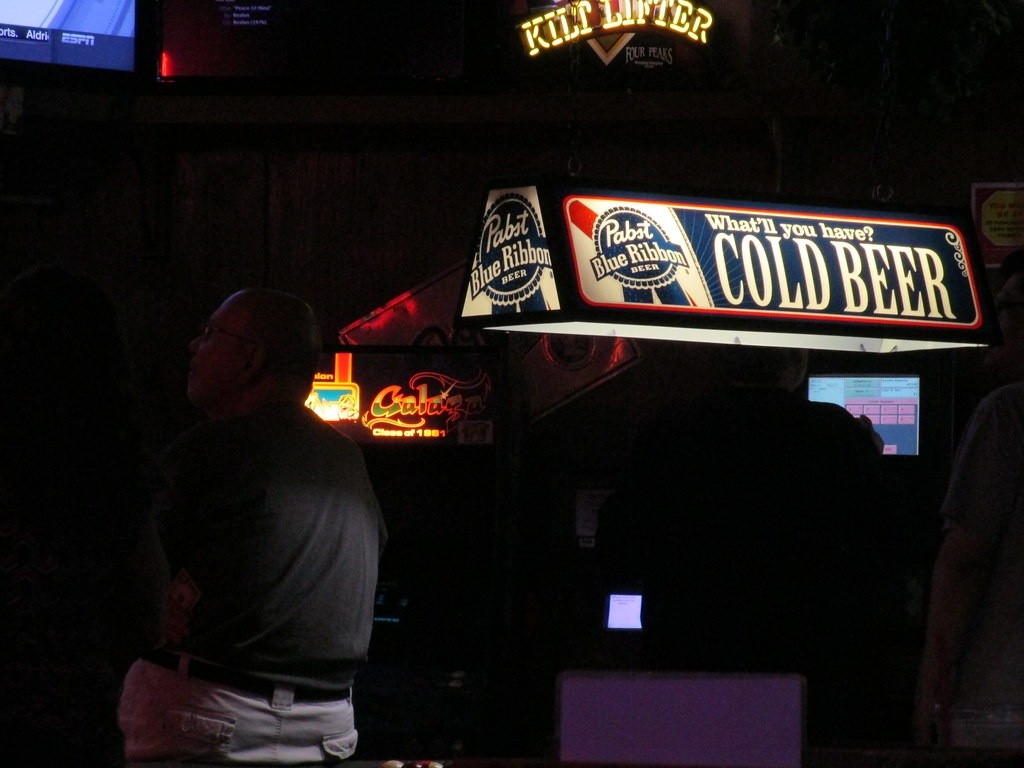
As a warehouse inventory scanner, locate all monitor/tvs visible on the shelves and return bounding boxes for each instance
[808,374,924,459]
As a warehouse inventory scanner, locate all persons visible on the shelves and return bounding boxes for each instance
[114,286,390,763]
[909,246,1024,751]
[585,346,894,746]
[0,262,170,768]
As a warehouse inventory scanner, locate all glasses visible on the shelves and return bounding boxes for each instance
[198,321,264,349]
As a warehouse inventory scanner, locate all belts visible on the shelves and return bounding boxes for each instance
[140,646,350,702]
